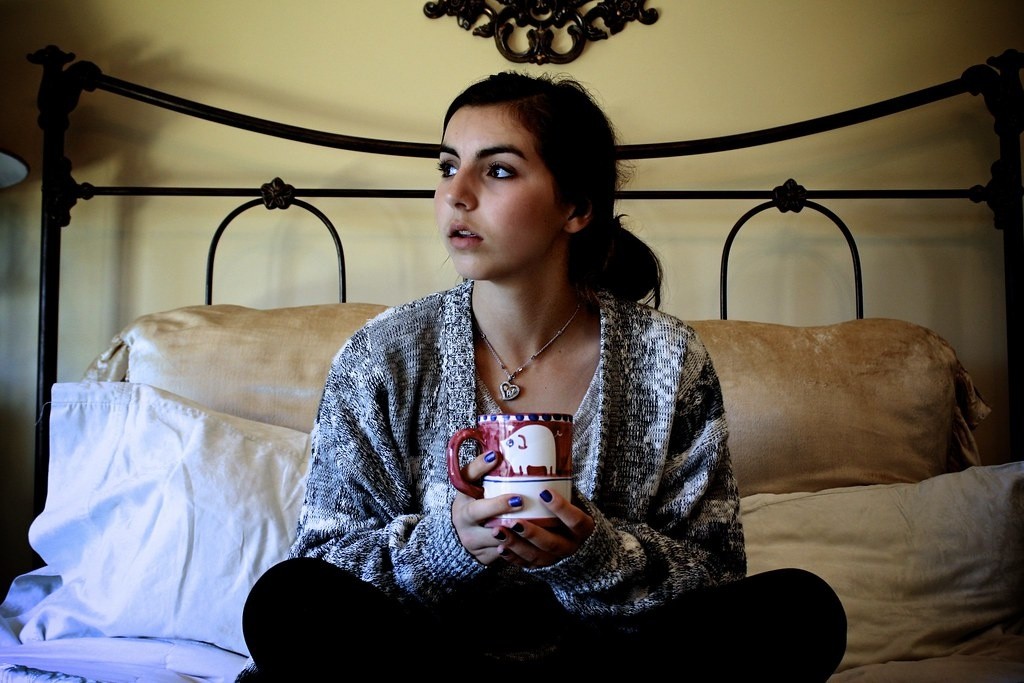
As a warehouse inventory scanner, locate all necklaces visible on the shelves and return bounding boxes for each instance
[474,303,580,400]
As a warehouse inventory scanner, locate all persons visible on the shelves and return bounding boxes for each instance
[241,72,847,683]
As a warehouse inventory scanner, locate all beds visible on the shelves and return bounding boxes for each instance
[0,44,1024,683]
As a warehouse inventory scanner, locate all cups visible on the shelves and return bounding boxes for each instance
[447,413,573,528]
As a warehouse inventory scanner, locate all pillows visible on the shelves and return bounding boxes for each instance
[76,302,993,509]
[27,381,1024,674]
